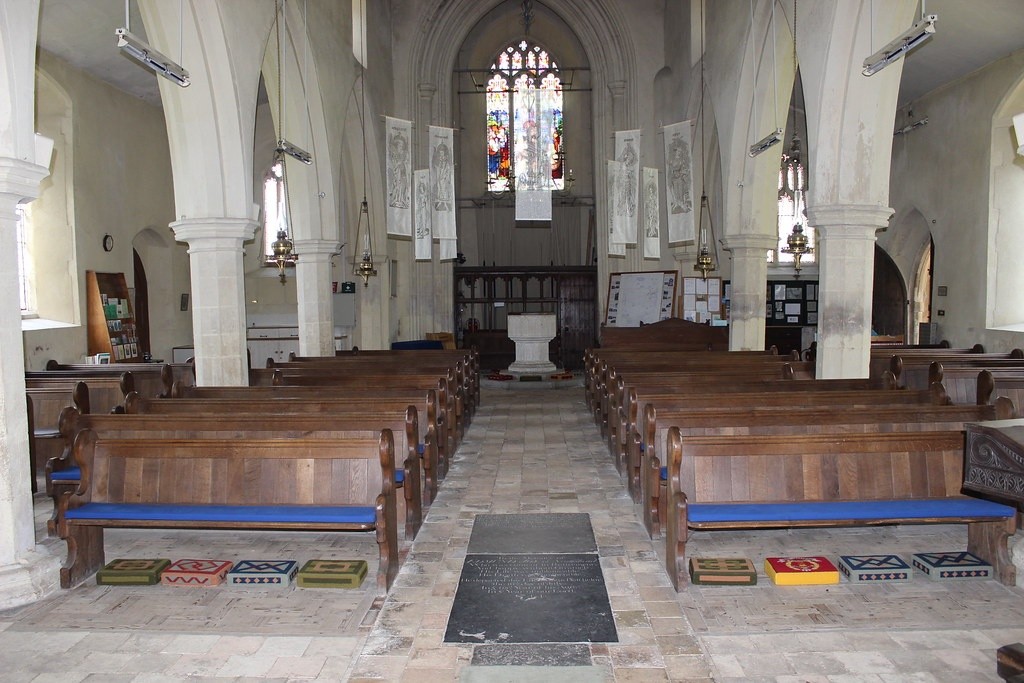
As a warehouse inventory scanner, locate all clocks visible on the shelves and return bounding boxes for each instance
[103,235,113,251]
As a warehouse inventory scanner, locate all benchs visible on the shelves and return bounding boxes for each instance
[23,345,479,597]
[585,316,1024,593]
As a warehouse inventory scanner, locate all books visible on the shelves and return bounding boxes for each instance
[85,352,111,365]
[101,294,137,360]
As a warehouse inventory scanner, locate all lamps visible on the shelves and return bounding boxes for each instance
[780,0,813,274]
[748,0,784,158]
[278,0,312,165]
[693,0,718,278]
[862,0,937,76]
[257,0,298,278]
[351,0,377,288]
[115,0,192,88]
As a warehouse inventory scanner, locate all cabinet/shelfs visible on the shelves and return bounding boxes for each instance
[86,270,142,363]
